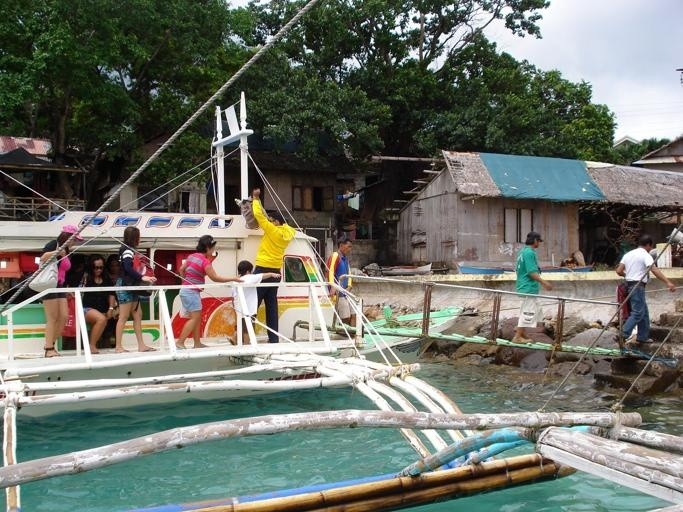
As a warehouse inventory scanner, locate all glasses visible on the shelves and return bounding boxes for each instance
[95,266,104,269]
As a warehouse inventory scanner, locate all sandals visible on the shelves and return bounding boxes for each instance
[613,334,626,345]
[44,342,61,357]
[636,338,653,343]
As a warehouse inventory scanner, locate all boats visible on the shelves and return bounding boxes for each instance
[360,261,433,277]
[454,262,595,274]
[0,90,462,423]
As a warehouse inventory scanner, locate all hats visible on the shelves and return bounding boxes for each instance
[62,224,84,241]
[527,232,543,242]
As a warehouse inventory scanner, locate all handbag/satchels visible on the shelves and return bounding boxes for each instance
[60,296,76,337]
[29,257,58,292]
[121,267,152,296]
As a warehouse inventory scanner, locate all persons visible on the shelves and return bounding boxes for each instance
[225,259,281,346]
[614,234,675,342]
[324,237,357,337]
[227,184,294,345]
[173,236,244,350]
[26,224,159,358]
[510,231,552,345]
